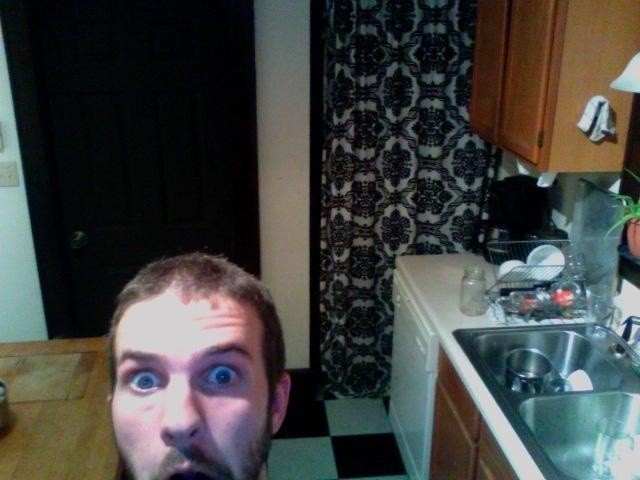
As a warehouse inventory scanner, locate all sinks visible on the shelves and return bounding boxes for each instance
[492,391,640,480]
[452,320,640,395]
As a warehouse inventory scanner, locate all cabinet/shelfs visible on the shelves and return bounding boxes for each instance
[467,1,640,175]
[386,251,640,480]
[478,236,603,327]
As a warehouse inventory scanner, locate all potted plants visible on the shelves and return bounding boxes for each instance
[599,165,640,261]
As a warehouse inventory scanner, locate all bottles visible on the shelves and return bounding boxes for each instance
[459,266,488,317]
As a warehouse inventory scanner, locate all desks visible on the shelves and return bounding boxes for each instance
[0,336,124,478]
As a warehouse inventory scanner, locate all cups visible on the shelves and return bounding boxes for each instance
[502,347,552,395]
[593,416,636,479]
[585,283,619,340]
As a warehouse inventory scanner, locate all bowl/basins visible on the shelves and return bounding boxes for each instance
[496,259,528,283]
[526,244,566,281]
[563,368,593,391]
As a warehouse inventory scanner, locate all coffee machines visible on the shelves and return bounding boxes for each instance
[471,174,569,265]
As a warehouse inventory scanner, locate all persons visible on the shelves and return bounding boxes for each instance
[102,250,294,480]
